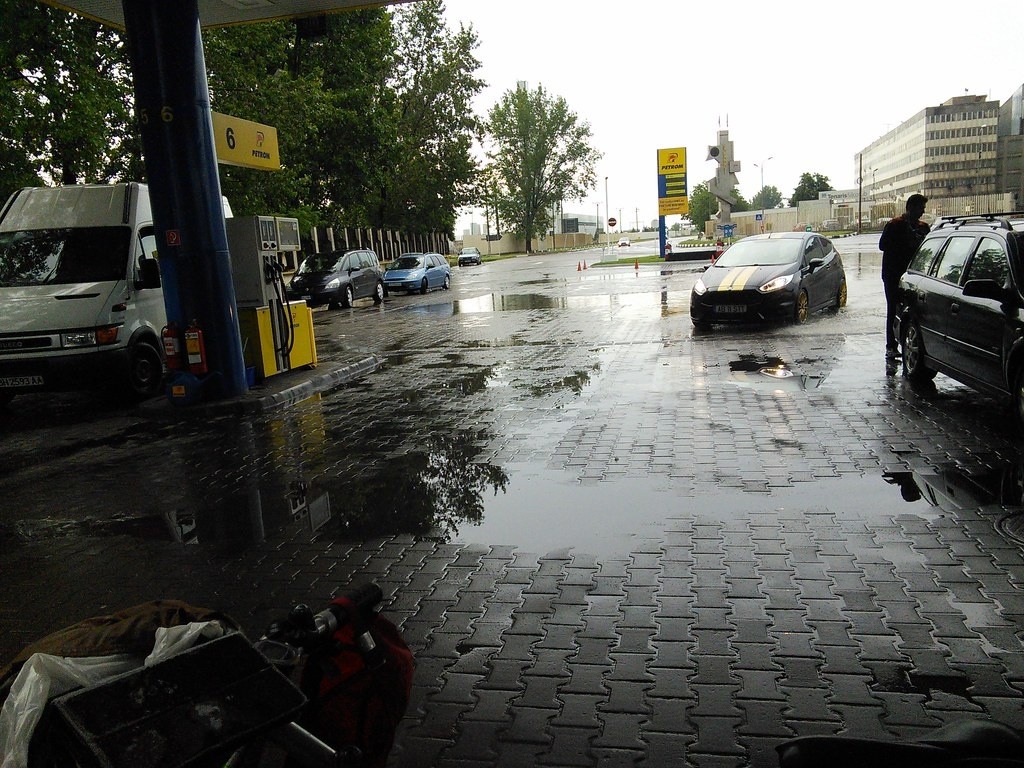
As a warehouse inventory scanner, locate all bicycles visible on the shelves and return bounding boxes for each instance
[0,580,1024,767]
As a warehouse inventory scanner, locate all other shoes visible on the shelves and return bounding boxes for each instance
[885,347,903,357]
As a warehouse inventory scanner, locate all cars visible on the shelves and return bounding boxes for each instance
[690,230,849,332]
[821,220,841,230]
[618,237,631,247]
[457,246,482,267]
[794,223,810,233]
[382,252,451,295]
[851,217,872,228]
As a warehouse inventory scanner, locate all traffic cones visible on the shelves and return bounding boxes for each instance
[583,259,588,270]
[576,262,582,271]
[710,255,715,264]
[633,259,638,270]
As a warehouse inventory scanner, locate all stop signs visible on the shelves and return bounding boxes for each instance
[608,218,617,226]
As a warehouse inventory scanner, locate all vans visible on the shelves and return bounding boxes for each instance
[285,249,387,310]
[0,183,234,396]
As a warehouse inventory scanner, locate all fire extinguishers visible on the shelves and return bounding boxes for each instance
[184,317,208,374]
[160,321,182,369]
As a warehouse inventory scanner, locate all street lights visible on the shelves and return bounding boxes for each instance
[976,124,988,216]
[873,168,879,203]
[604,177,609,250]
[753,155,774,233]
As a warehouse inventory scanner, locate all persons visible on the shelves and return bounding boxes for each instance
[879,194,931,357]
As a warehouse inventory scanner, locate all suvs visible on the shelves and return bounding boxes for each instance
[886,209,1024,424]
